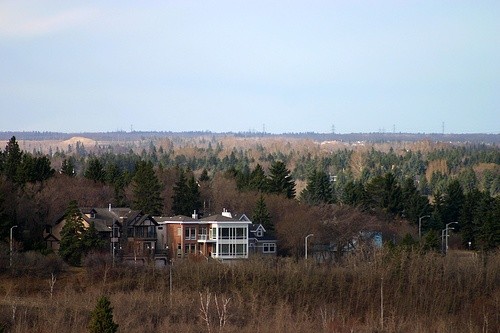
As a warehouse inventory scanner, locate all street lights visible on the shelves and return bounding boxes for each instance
[9,225,19,266]
[305,233,315,259]
[200,250,209,262]
[442,221,458,252]
[419,216,430,238]
[112,247,117,267]
